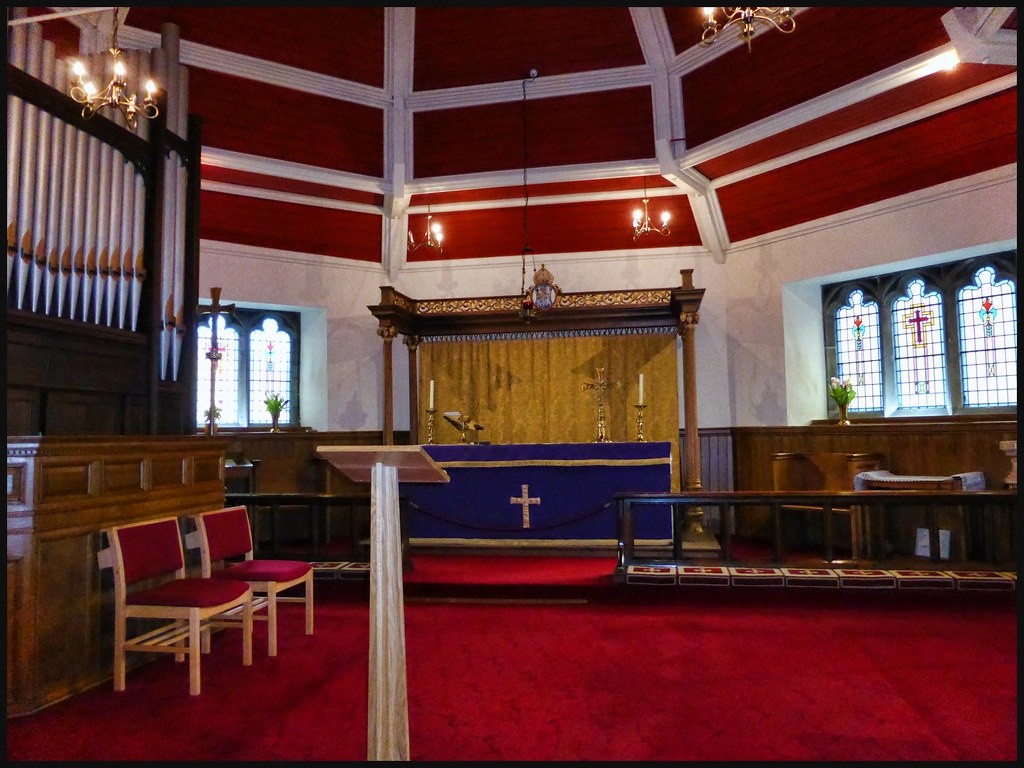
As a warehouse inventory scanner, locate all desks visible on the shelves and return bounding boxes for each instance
[614,489,1018,590]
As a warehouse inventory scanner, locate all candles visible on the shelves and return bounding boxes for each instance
[639,374,644,404]
[430,380,434,408]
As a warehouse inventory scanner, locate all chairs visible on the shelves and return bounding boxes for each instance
[184,505,314,656]
[98,515,253,696]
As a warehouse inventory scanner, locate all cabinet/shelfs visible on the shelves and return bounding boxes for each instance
[9,434,238,718]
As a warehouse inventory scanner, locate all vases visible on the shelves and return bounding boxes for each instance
[839,404,850,426]
[271,414,280,433]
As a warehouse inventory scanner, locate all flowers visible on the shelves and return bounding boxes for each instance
[829,376,856,406]
[264,393,285,414]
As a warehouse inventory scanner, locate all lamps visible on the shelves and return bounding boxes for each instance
[69,5,159,129]
[406,202,444,253]
[632,176,671,241]
[701,6,796,54]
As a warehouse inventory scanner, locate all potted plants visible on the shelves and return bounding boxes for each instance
[204,405,222,434]
